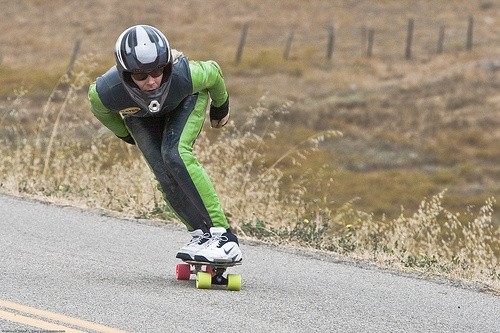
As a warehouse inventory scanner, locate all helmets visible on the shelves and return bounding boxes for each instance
[115,25,173,114]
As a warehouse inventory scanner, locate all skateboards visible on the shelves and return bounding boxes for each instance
[175,259,243,291]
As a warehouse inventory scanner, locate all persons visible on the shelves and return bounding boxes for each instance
[86,25,244,264]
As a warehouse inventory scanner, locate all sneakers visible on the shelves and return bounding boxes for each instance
[194,227,242,262]
[176,229,213,261]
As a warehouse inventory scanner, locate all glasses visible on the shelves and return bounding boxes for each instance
[131,67,164,81]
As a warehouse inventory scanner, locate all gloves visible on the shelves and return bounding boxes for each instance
[115,133,135,146]
[210,101,230,129]
